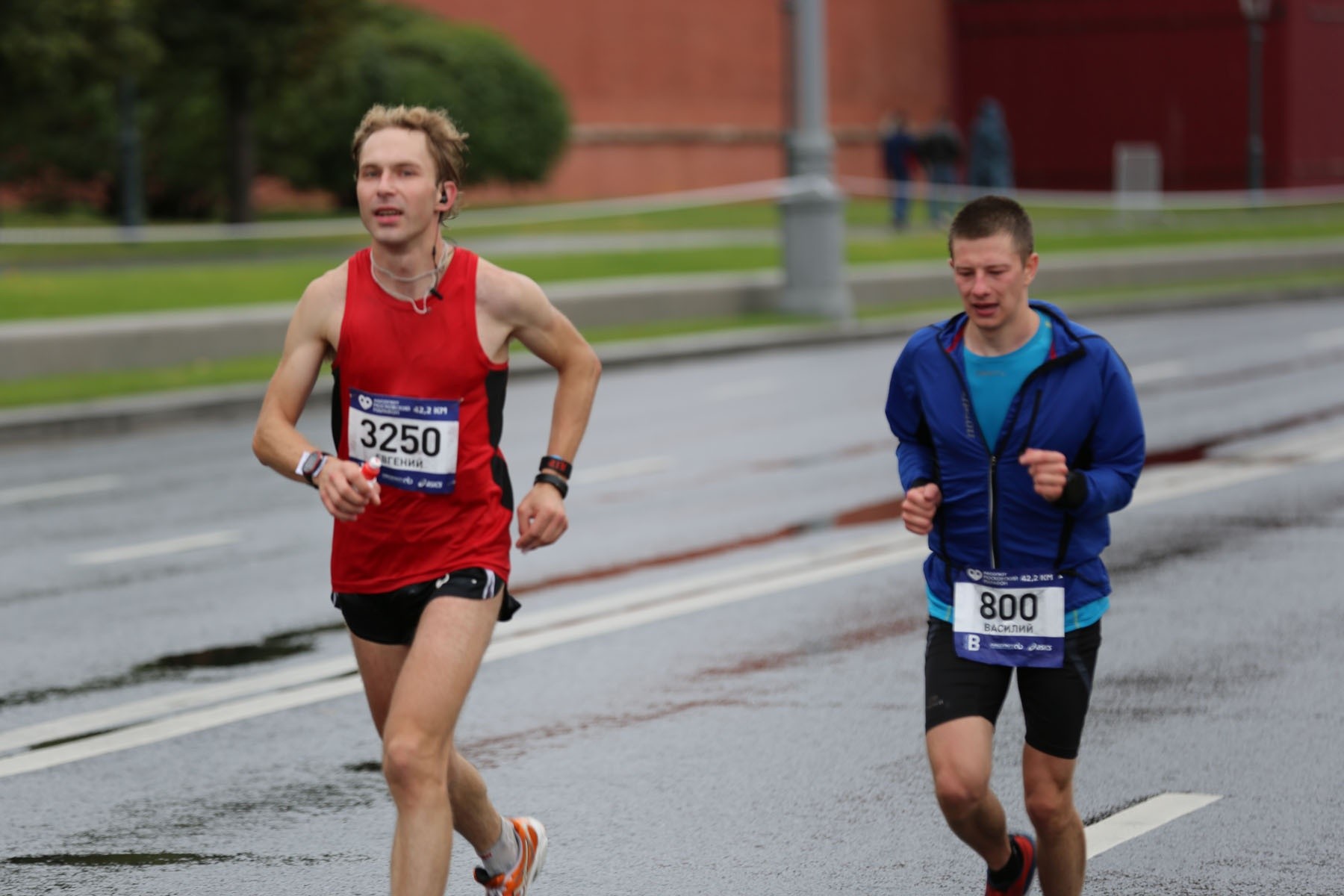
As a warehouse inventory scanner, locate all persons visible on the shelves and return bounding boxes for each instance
[252,103,602,896]
[882,195,1148,896]
[878,106,1011,230]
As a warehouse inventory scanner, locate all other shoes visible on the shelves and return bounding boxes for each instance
[984,833,1036,896]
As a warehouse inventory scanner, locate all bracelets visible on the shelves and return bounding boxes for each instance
[539,456,572,479]
[533,474,568,498]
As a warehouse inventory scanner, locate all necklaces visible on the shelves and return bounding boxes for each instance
[368,243,450,282]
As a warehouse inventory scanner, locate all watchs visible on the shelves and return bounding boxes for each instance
[303,451,334,490]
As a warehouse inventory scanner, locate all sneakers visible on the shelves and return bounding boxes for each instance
[472,815,549,896]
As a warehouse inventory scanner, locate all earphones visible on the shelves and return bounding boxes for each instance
[441,191,448,204]
